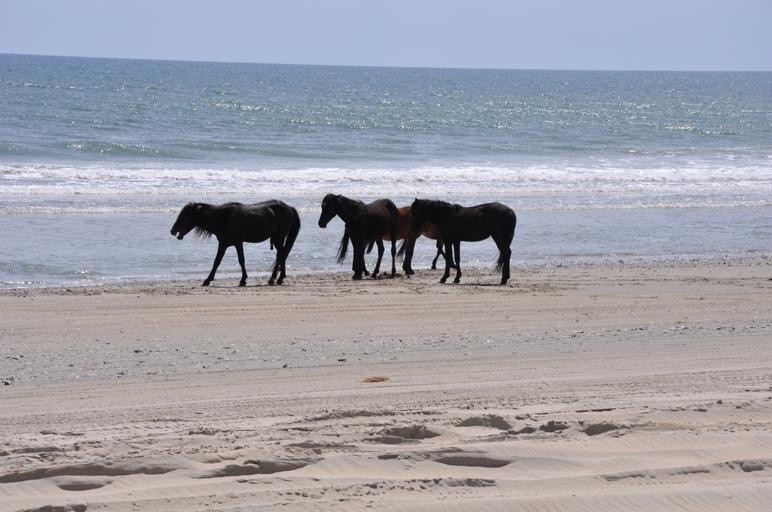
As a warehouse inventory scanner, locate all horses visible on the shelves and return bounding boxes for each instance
[318,194,517,285]
[170,199,301,287]
